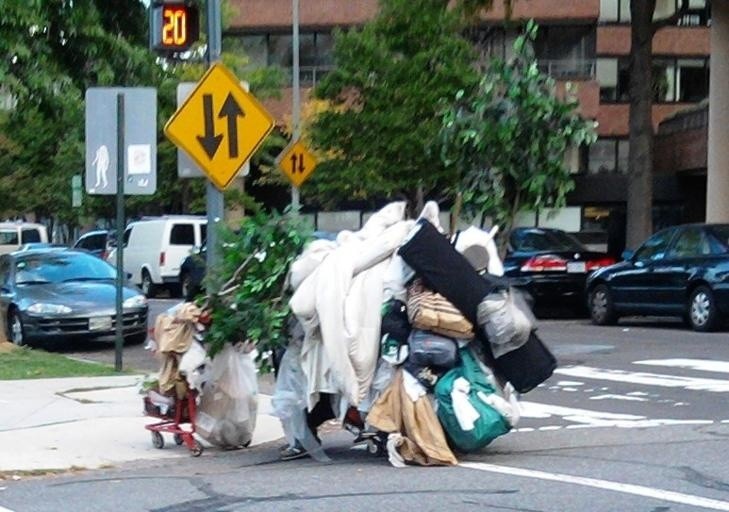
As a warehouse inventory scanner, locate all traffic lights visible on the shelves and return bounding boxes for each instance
[146,0,201,54]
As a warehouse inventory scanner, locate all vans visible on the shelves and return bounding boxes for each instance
[108,215,211,298]
[0,220,51,253]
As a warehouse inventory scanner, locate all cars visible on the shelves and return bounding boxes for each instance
[0,243,149,352]
[70,230,109,258]
[582,223,728,331]
[495,226,615,317]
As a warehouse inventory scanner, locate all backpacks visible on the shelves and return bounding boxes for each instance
[406,277,475,340]
[408,328,457,366]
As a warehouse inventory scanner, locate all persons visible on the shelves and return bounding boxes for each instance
[274,435,323,458]
